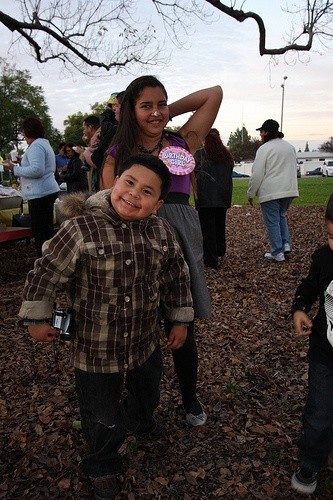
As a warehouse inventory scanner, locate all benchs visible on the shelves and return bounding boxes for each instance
[0,226,32,243]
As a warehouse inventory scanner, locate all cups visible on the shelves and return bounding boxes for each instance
[11,152,18,161]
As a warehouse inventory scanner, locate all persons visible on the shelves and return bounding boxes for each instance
[8,117,60,258]
[17,151,193,500]
[56,142,71,185]
[190,128,235,268]
[57,143,89,192]
[290,194,333,494]
[84,92,126,195]
[72,115,102,197]
[247,119,299,261]
[102,75,223,426]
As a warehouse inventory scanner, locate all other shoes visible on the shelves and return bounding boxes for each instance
[181,398,207,426]
[290,463,318,495]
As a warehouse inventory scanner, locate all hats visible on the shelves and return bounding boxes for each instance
[108,92,118,104]
[255,119,279,132]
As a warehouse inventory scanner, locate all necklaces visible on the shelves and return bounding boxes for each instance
[137,135,163,154]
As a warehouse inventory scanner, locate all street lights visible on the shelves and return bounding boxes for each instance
[281,76,288,133]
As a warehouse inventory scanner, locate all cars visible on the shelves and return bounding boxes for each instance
[296,159,333,178]
[232,171,250,178]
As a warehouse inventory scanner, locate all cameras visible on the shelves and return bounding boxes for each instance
[49,307,81,341]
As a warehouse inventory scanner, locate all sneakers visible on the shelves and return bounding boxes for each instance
[284,244,291,254]
[264,252,285,262]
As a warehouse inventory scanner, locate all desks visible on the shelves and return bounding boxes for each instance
[0,195,22,210]
